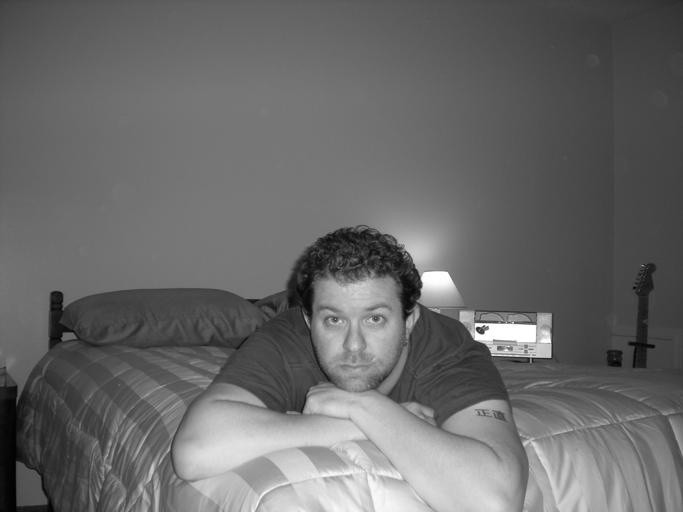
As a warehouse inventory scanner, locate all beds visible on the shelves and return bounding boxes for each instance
[47,290,683,512]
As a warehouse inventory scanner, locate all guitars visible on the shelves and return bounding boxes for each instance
[633,263,656,367]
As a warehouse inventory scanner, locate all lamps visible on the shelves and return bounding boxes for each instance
[415,271,465,314]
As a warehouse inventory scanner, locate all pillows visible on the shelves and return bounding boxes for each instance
[253,289,301,322]
[59,288,275,349]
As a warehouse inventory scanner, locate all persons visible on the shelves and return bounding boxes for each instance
[169,222,530,511]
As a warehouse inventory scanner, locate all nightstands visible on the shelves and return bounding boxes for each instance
[0,373,18,512]
